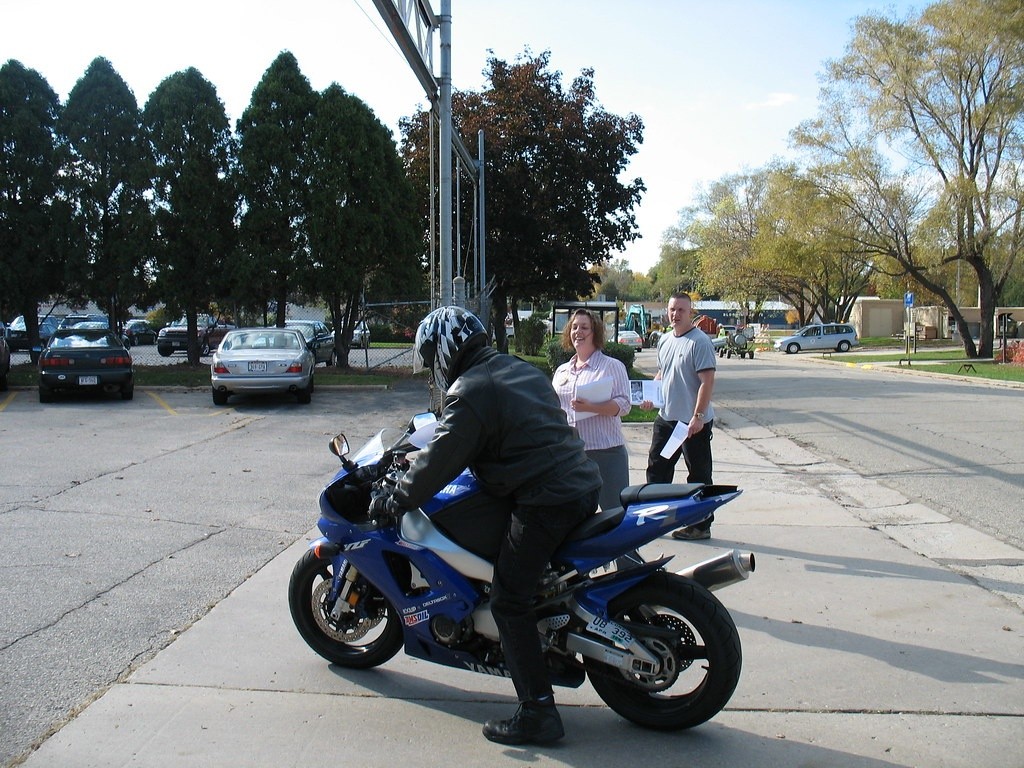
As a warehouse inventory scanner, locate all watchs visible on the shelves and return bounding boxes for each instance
[693,411,705,419]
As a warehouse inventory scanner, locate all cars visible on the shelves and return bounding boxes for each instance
[0,322,10,392]
[773,322,859,352]
[32,327,134,401]
[71,323,129,349]
[118,321,158,347]
[210,328,319,406]
[609,330,642,352]
[253,320,337,367]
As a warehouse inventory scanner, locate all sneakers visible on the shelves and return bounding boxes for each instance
[672,526,711,540]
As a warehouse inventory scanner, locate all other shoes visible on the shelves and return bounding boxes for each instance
[482,696,564,745]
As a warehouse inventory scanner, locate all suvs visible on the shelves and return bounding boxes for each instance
[331,321,371,348]
[59,313,109,328]
[7,314,58,351]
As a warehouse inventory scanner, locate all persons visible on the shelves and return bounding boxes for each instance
[368,303,606,749]
[639,293,716,539]
[260,317,286,349]
[549,308,633,513]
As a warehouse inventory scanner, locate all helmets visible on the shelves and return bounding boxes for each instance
[413,306,488,393]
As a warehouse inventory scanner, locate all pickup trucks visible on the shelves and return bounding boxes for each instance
[493,311,548,341]
[155,313,237,357]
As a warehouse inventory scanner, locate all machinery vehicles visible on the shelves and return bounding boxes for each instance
[713,325,738,351]
[645,313,666,343]
[613,305,653,347]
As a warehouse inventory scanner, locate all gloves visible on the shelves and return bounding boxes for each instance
[368,495,406,528]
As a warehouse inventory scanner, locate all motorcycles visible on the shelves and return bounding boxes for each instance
[288,408,755,732]
